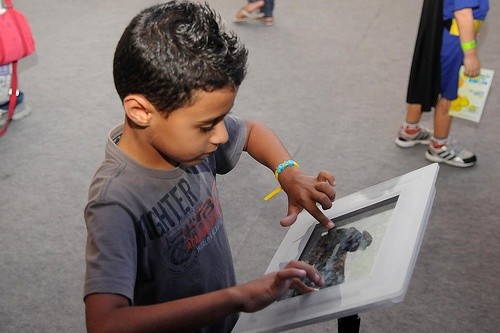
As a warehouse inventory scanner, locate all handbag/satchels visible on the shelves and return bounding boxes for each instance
[0,0,34,66]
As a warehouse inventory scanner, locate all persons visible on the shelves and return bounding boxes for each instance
[232,0,276,27]
[0,0,32,129]
[82,0,336,333]
[395,0,491,168]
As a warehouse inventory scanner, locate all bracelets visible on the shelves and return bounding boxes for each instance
[460,39,477,52]
[263,159,301,201]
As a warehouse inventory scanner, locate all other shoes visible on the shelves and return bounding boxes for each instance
[0,100,30,125]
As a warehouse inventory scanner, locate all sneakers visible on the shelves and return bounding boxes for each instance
[424,140,477,167]
[395,125,434,148]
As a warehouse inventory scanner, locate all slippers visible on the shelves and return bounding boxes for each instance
[255,14,272,26]
[234,9,265,23]
